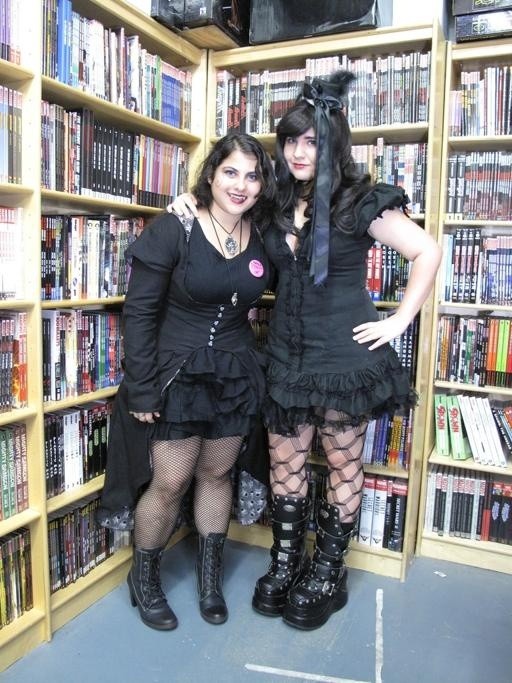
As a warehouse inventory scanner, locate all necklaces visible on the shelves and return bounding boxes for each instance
[204,206,243,308]
[209,206,240,256]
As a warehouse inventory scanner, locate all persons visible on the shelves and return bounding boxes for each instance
[120,132,277,633]
[163,71,446,636]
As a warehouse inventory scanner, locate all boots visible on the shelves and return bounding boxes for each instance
[128,548,178,630]
[284,503,350,630]
[253,498,312,617]
[196,533,228,624]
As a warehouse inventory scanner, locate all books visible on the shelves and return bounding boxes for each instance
[371,474,389,548]
[43,97,190,214]
[45,491,132,599]
[41,211,154,300]
[447,65,512,137]
[452,0,512,15]
[307,468,328,530]
[1,528,35,628]
[388,477,408,553]
[43,305,127,404]
[270,137,429,214]
[348,48,431,127]
[309,430,326,457]
[383,476,395,548]
[432,393,512,473]
[42,400,113,500]
[359,473,376,546]
[423,461,512,546]
[1,1,22,66]
[446,150,512,222]
[1,424,30,521]
[456,10,512,44]
[1,207,27,299]
[1,85,23,185]
[442,227,512,308]
[360,407,413,471]
[215,70,306,135]
[366,235,414,302]
[41,0,191,132]
[435,312,511,388]
[377,308,419,389]
[306,55,347,78]
[0,309,31,414]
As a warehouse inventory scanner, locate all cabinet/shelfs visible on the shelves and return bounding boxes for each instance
[0,0,53,674]
[415,41,512,574]
[40,0,206,634]
[205,25,436,582]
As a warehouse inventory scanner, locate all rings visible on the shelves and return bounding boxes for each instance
[136,414,145,418]
